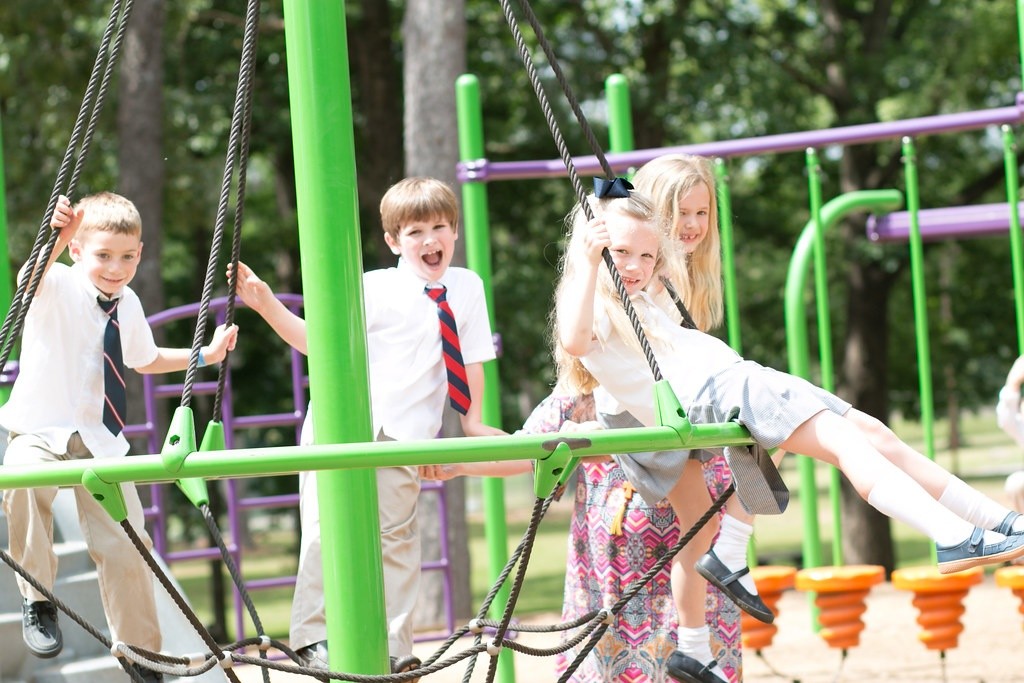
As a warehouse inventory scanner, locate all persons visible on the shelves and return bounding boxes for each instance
[561,151,770,682]
[0,183,240,682]
[832,213,898,358]
[560,194,1024,566]
[224,171,526,683]
[938,320,975,380]
[994,322,1024,538]
[418,288,742,682]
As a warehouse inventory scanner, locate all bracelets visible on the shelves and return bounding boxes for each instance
[192,347,207,368]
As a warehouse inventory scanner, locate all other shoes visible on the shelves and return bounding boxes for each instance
[667,650,729,683]
[934,509,1024,575]
[693,548,774,625]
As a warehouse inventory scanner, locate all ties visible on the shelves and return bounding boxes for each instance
[96,295,126,438]
[423,284,472,416]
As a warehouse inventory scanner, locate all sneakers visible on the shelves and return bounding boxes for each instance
[20,597,64,658]
[117,656,164,683]
